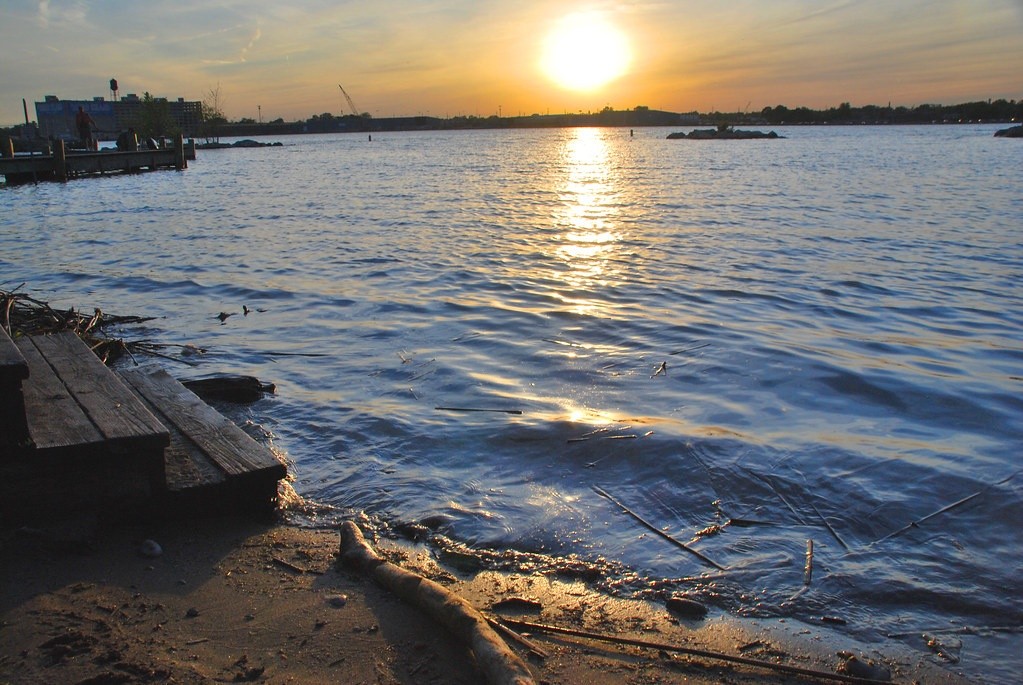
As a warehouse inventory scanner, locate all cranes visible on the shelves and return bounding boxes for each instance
[336,83,359,117]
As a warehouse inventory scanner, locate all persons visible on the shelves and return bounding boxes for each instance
[76,106,98,151]
